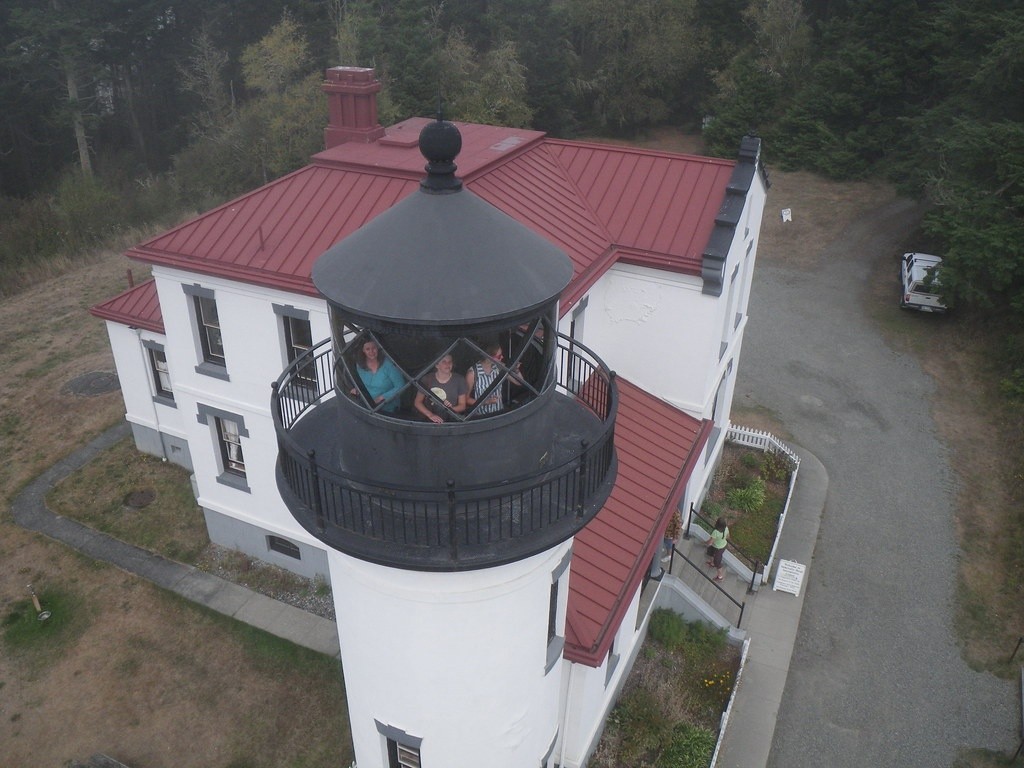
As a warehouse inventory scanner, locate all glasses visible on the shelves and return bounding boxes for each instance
[492,353,504,359]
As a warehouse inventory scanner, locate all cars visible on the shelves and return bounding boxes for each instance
[900,250,951,315]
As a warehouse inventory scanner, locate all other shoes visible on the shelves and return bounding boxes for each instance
[705,561,714,568]
[712,576,723,582]
[661,555,671,562]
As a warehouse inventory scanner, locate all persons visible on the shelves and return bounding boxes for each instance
[413,352,468,423]
[349,335,405,413]
[464,343,523,416]
[660,508,681,562]
[701,517,730,581]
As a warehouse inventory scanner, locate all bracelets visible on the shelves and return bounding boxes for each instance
[516,370,520,374]
